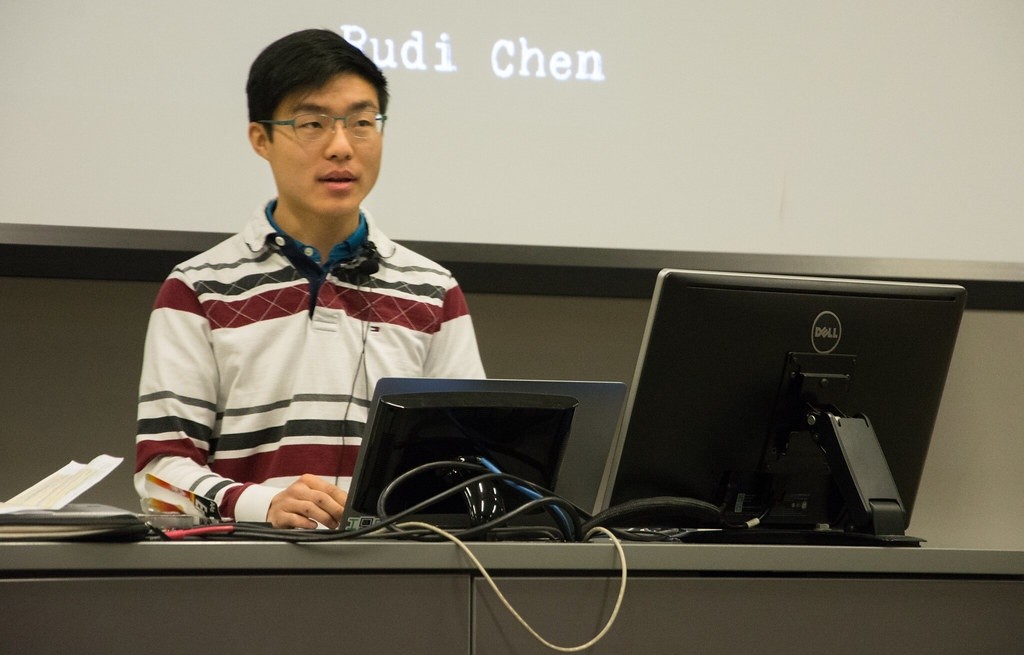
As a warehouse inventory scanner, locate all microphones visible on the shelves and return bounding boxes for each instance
[360,249,379,274]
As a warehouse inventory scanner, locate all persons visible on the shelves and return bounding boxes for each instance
[133,26,491,534]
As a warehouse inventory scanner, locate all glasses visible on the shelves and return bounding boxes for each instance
[254,111,387,143]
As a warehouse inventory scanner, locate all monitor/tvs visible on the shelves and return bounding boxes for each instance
[338,378,626,534]
[589,269,967,537]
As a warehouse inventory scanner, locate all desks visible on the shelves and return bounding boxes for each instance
[1,520,1023,654]
